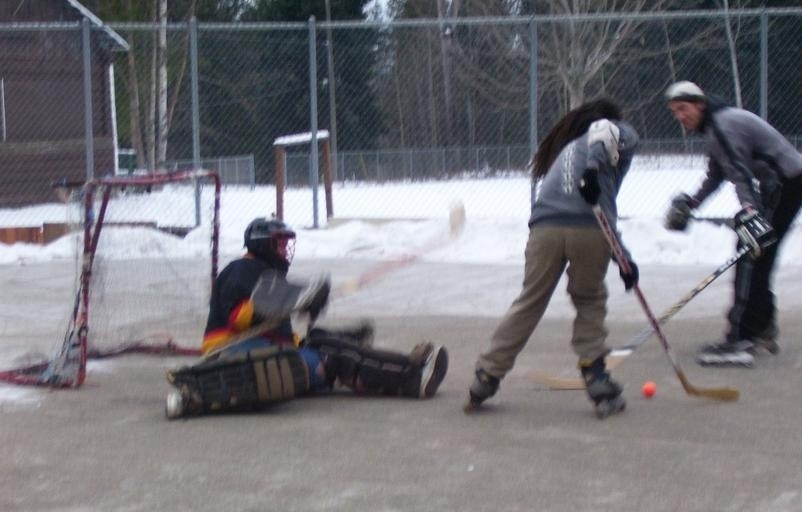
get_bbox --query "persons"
[166,212,449,419]
[661,80,802,367]
[465,98,641,419]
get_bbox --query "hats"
[664,81,705,105]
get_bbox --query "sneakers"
[166,392,184,418]
[419,344,448,398]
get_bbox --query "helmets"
[244,218,296,271]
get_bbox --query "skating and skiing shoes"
[695,338,755,368]
[465,369,499,410]
[749,326,781,353]
[581,357,625,418]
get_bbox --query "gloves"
[578,169,601,204]
[735,206,778,260]
[665,194,692,230]
[619,261,638,289]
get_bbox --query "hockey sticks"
[581,179,739,401]
[192,207,464,368]
[550,245,751,390]
